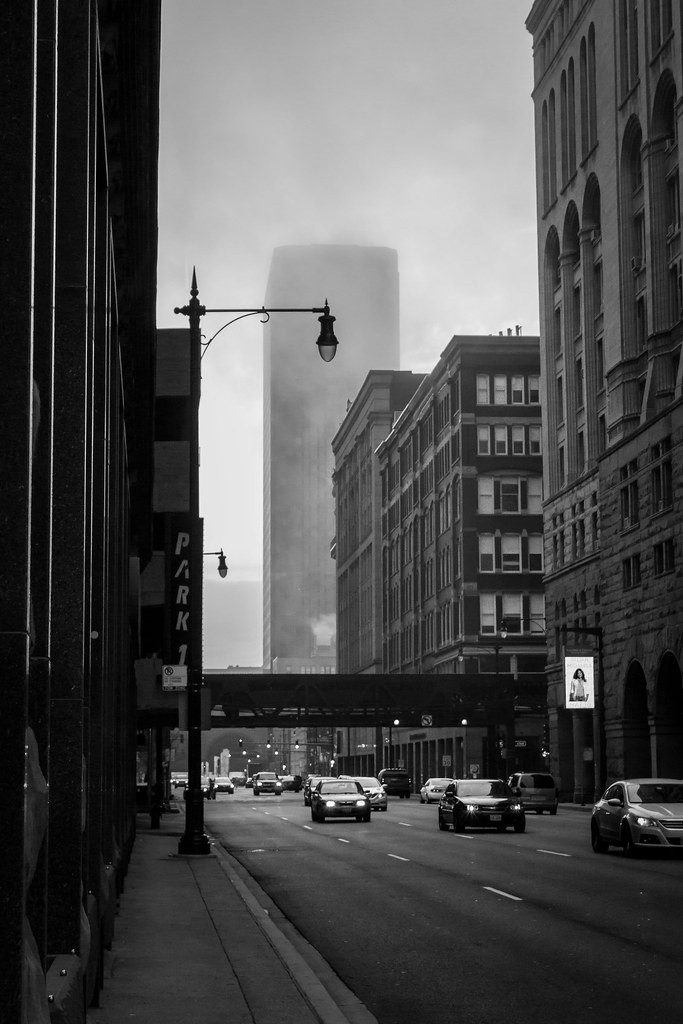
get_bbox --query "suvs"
[505,770,559,816]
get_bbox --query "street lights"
[455,642,508,779]
[178,265,342,853]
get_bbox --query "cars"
[438,777,526,834]
[589,777,683,857]
[171,767,456,823]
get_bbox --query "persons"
[570,669,590,701]
